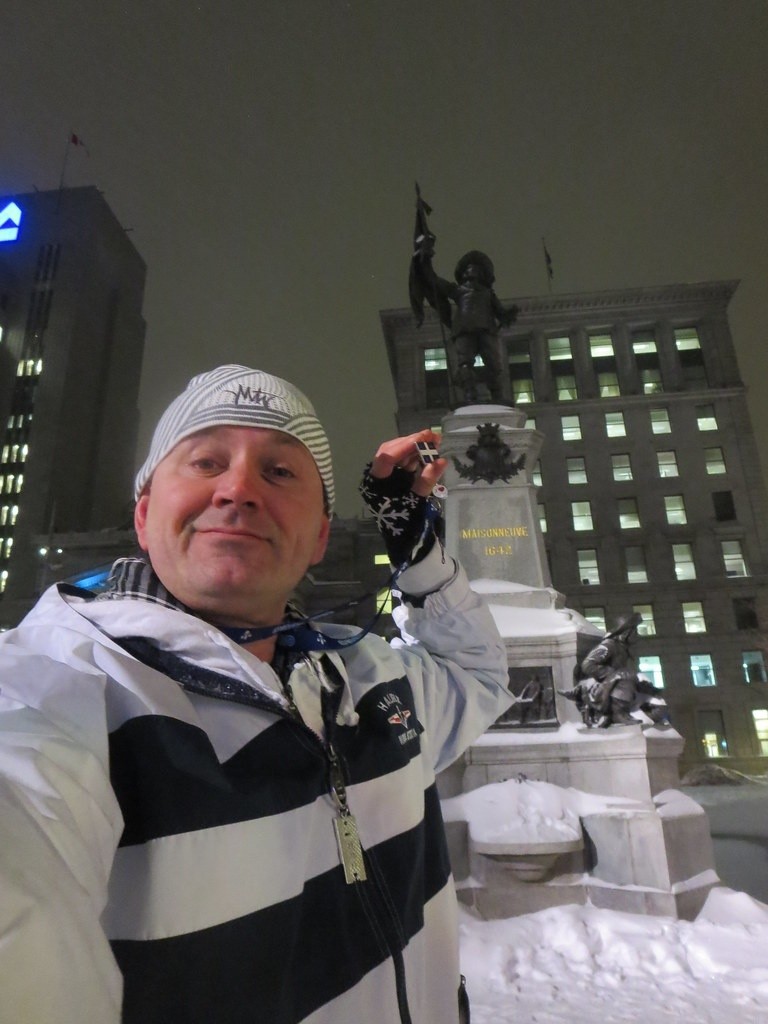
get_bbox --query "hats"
[137,365,337,525]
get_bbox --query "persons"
[0,364,517,1024]
[518,673,542,725]
[578,612,668,727]
[413,232,520,405]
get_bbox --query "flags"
[543,249,554,279]
